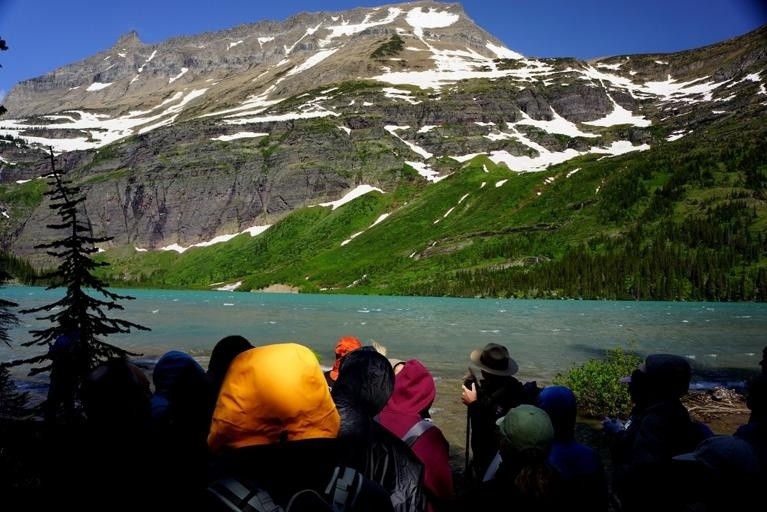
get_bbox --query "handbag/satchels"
[283,444,399,510]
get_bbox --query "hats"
[470,342,518,377]
[496,404,553,449]
[620,355,692,397]
[672,434,755,476]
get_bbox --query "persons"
[670,433,764,510]
[462,340,601,511]
[742,340,766,436]
[71,334,458,512]
[602,355,710,479]
